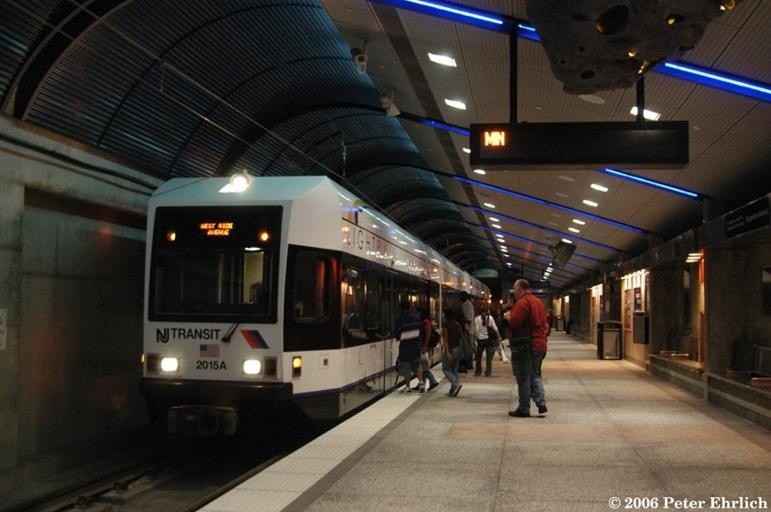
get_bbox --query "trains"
[142,169,492,435]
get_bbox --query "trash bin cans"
[597,321,622,360]
[556,316,564,331]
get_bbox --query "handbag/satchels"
[511,336,532,363]
[487,314,498,339]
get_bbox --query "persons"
[389,286,512,398]
[503,279,548,417]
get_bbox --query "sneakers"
[399,364,550,418]
